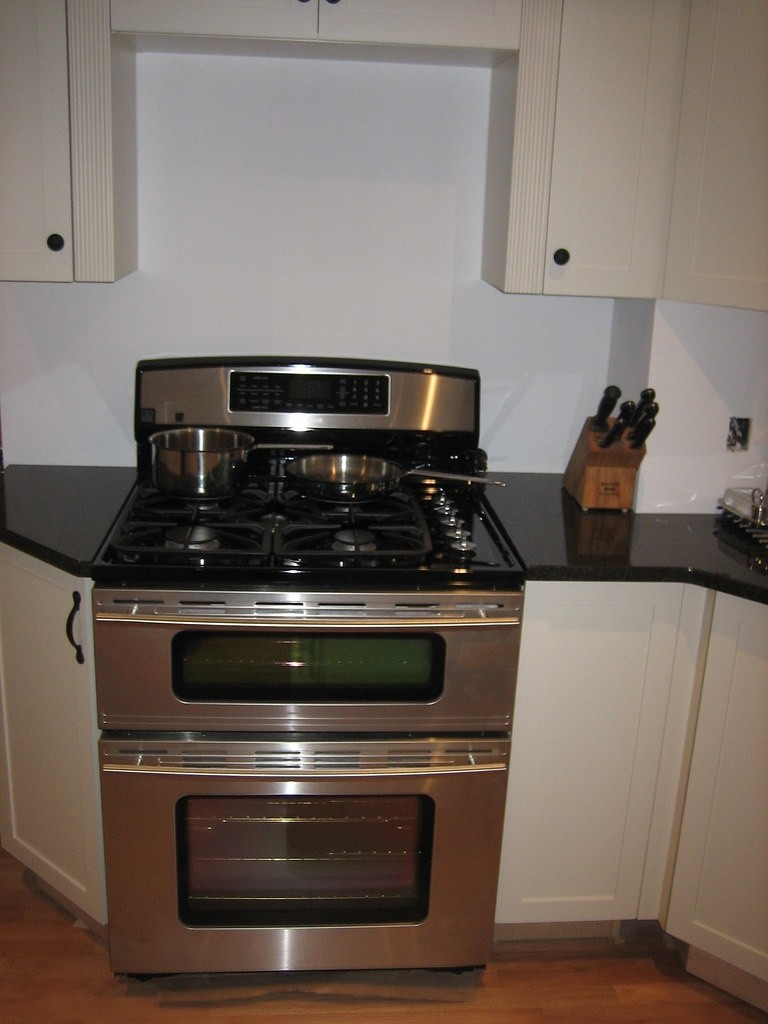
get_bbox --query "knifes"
[588,382,661,451]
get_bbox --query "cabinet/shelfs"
[0,536,111,944]
[1,0,140,286]
[480,0,768,316]
[109,0,521,71]
[494,580,768,1017]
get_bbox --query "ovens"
[94,593,524,975]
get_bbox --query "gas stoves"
[93,349,524,601]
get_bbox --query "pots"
[146,426,334,502]
[280,449,509,511]
